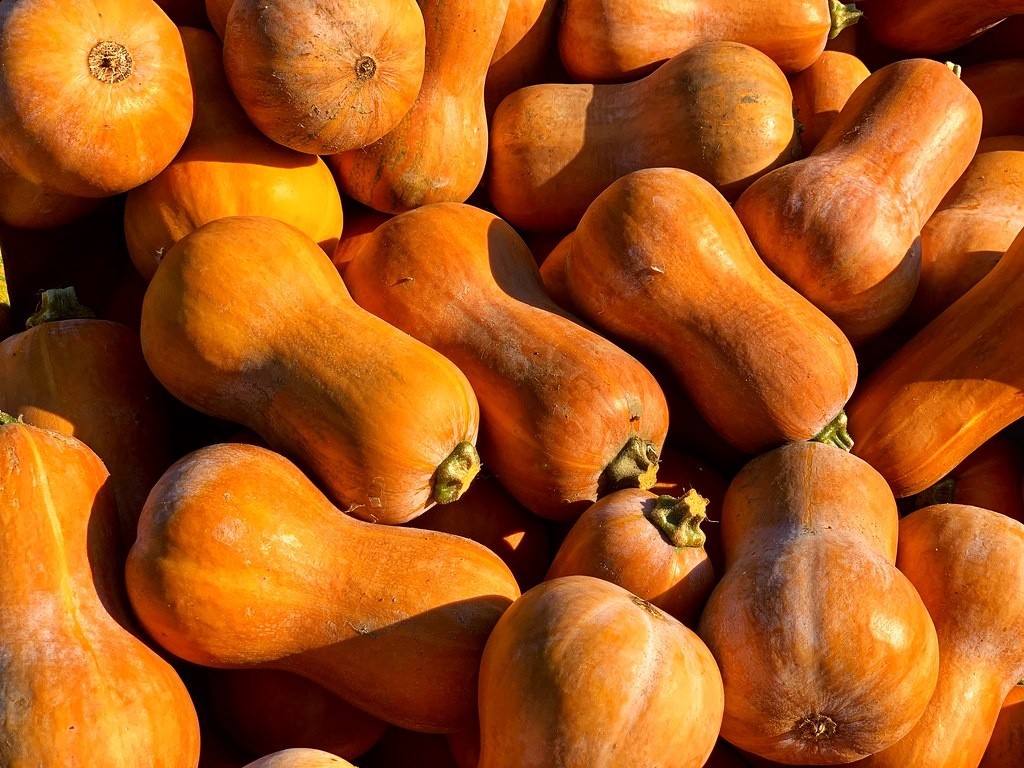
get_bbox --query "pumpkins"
[0,0,1024,768]
